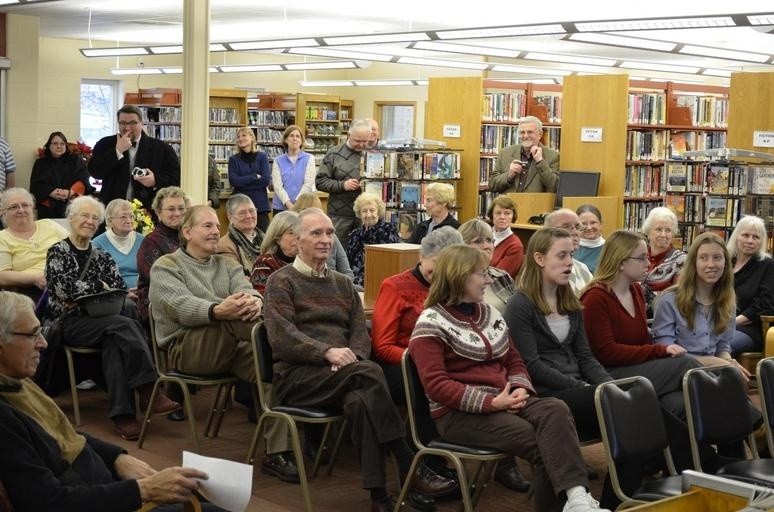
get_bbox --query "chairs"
[394,344,533,511]
[591,376,690,507]
[246,317,347,509]
[684,365,774,485]
[139,302,263,457]
[51,303,773,459]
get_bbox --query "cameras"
[517,161,529,175]
[131,167,147,179]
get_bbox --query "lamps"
[79,5,772,56]
[110,49,732,86]
[562,32,774,64]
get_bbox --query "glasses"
[3,201,30,214]
[626,254,650,264]
[554,224,584,235]
[118,119,139,128]
[110,213,136,223]
[5,325,45,343]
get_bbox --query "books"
[306,103,352,179]
[628,92,728,125]
[477,124,561,219]
[361,153,460,245]
[139,106,295,181]
[484,93,561,122]
[625,129,774,255]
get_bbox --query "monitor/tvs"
[553,170,601,210]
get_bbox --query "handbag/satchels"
[31,313,62,394]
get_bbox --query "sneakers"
[304,441,329,465]
[111,414,144,441]
[563,494,610,510]
[371,496,393,511]
[495,459,531,493]
[399,459,470,511]
[139,387,182,417]
[262,451,301,483]
[76,378,96,390]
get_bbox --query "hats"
[74,289,129,318]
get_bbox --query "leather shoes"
[168,411,185,421]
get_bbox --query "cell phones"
[358,176,366,181]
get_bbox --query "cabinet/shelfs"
[555,74,731,241]
[355,144,463,238]
[119,87,355,199]
[425,78,562,237]
[684,73,773,243]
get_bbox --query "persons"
[0,182,774,512]
[89,107,181,224]
[228,127,271,235]
[316,120,379,249]
[30,132,90,220]
[206,154,221,210]
[489,117,559,193]
[271,125,317,218]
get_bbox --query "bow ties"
[131,141,136,146]
